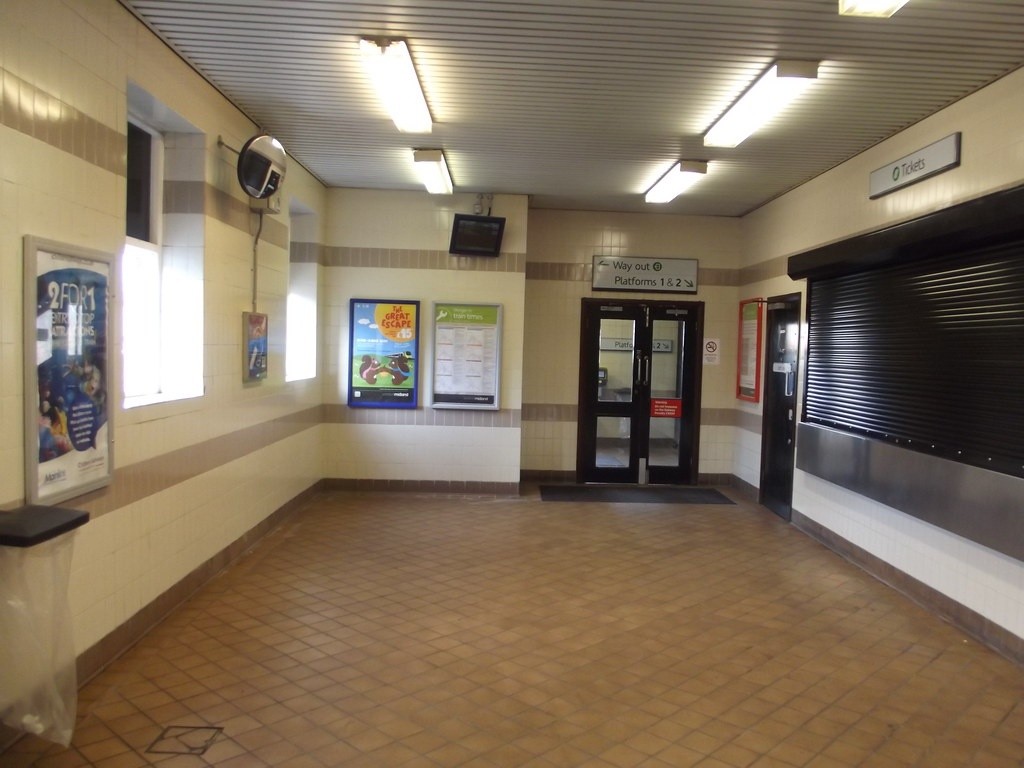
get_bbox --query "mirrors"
[236,133,289,200]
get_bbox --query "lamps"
[644,160,707,203]
[414,149,454,196]
[838,0,913,19]
[702,60,819,149]
[358,36,434,133]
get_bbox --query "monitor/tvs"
[449,213,506,257]
